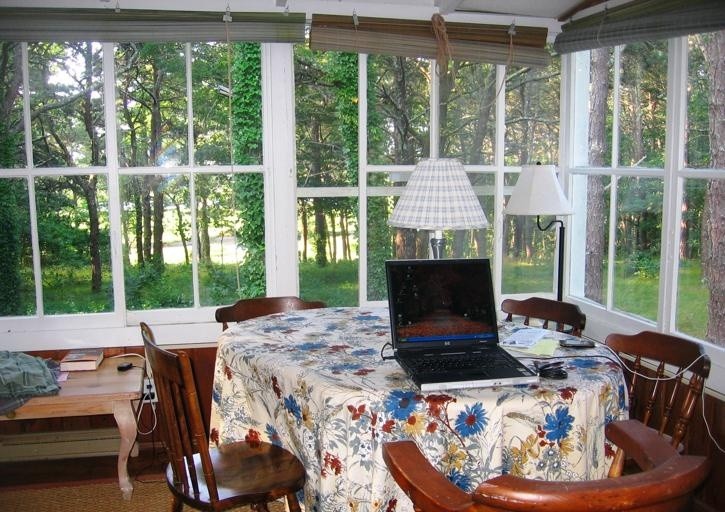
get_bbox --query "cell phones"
[117,361,132,371]
[559,339,595,348]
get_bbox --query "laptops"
[385,258,539,391]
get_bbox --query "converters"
[142,392,154,400]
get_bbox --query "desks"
[0,354,146,501]
[205,306,631,512]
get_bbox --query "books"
[59,349,104,370]
[499,328,548,348]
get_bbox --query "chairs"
[606,330,711,465]
[500,296,586,337]
[381,418,710,512]
[214,297,328,331]
[139,322,307,512]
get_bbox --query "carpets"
[0,476,290,512]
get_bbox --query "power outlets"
[142,376,159,403]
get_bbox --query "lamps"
[501,161,573,332]
[386,156,489,260]
